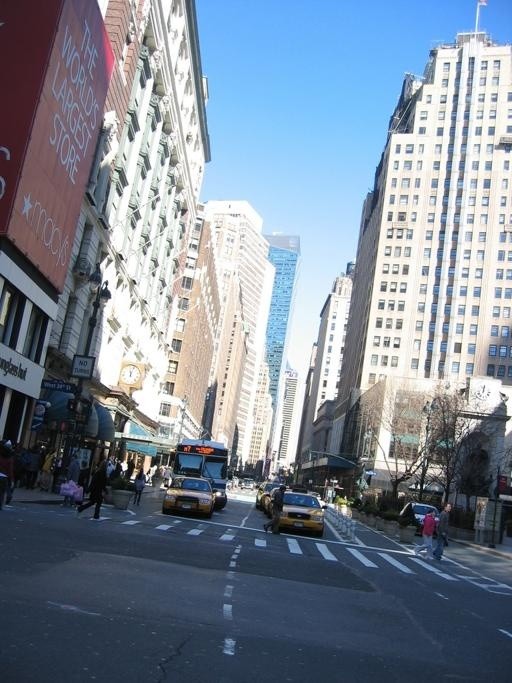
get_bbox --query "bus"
[170,438,228,511]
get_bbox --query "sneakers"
[75,507,80,520]
[94,517,104,522]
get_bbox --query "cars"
[233,476,255,491]
[163,476,217,519]
[400,501,440,538]
[256,481,327,537]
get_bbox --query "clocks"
[118,361,146,391]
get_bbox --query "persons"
[0,435,173,522]
[414,502,451,561]
[263,485,286,535]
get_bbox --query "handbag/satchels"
[60,480,84,502]
